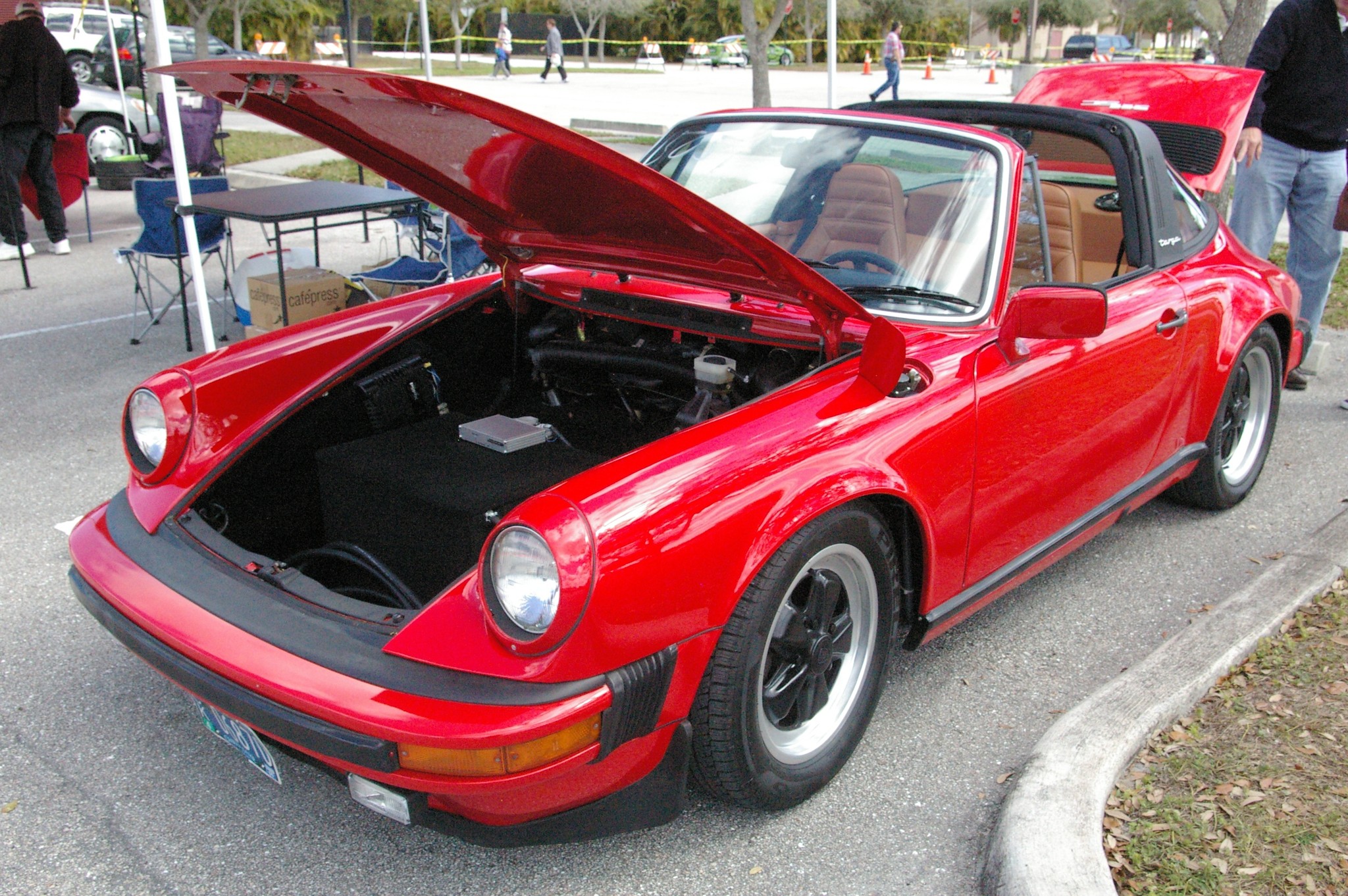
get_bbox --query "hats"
[15,0,44,18]
[891,20,901,31]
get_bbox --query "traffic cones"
[985,57,998,84]
[862,50,872,75]
[922,54,933,79]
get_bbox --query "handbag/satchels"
[502,43,513,54]
[550,53,561,68]
[1334,181,1348,231]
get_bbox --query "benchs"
[905,190,1134,282]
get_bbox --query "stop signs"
[1166,18,1173,32]
[1011,8,1020,24]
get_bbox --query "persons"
[0,0,80,261]
[538,19,568,83]
[1226,0,1348,390]
[491,21,513,80]
[868,21,904,102]
[1191,38,1206,63]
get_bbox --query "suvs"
[91,24,273,92]
[43,2,144,84]
[1063,34,1143,61]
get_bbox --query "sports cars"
[69,57,1314,846]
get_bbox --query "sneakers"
[0,241,35,260]
[47,238,71,254]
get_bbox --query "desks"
[163,180,426,352]
[19,133,94,244]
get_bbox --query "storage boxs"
[230,249,346,340]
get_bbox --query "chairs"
[113,176,240,346]
[1007,182,1084,304]
[795,163,908,276]
[140,91,230,179]
[350,179,497,302]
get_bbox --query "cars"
[708,34,794,67]
[57,80,162,176]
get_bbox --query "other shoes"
[869,94,875,101]
[1340,399,1348,409]
[1285,366,1308,388]
[488,76,497,79]
[536,78,544,82]
[560,78,569,82]
[503,75,512,80]
